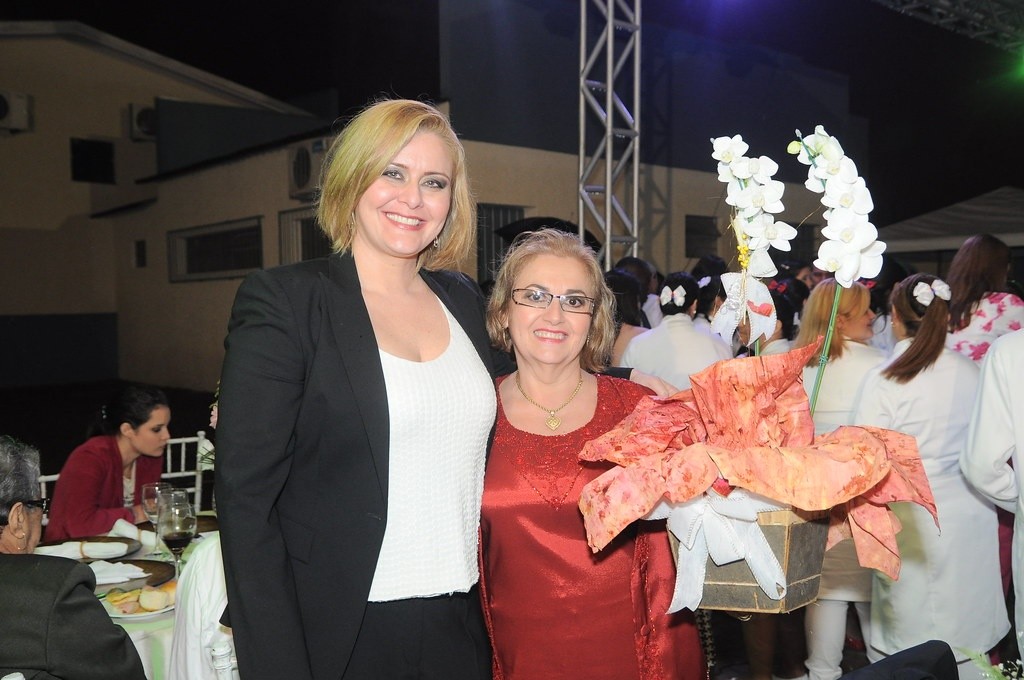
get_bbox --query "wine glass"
[141,482,172,560]
[157,489,197,583]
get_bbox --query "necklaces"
[513,370,583,431]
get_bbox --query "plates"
[98,596,175,620]
[36,536,142,564]
[86,559,175,596]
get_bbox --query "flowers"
[702,120,893,448]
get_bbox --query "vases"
[665,501,837,612]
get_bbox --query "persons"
[1,434,146,680]
[211,109,680,680]
[38,390,172,540]
[601,237,1024,680]
[481,229,703,680]
[961,327,1024,659]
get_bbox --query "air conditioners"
[0,88,29,129]
[130,102,159,141]
[286,138,329,197]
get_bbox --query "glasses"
[512,288,595,316]
[17,498,48,513]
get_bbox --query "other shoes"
[843,629,865,651]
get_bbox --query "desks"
[36,503,227,680]
